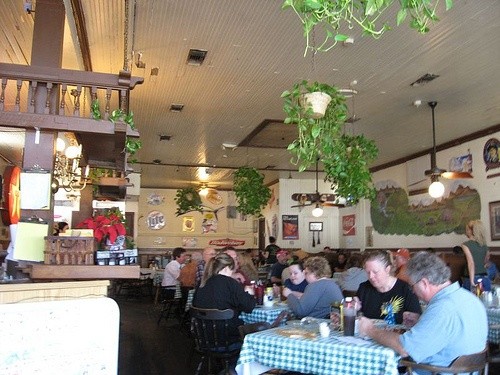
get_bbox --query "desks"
[238,290,295,331]
[234,317,414,375]
[127,265,158,298]
[149,268,166,302]
[483,304,500,344]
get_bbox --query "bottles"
[250,280,280,306]
[343,297,355,336]
[149,259,158,271]
[473,279,484,298]
[384,303,395,325]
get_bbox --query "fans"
[193,182,218,192]
[405,101,474,187]
[289,157,346,210]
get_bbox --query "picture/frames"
[489,200,500,241]
[309,221,323,232]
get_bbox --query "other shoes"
[220,369,236,375]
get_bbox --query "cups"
[481,291,492,307]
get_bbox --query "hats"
[392,249,410,257]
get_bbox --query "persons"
[262,236,280,264]
[193,253,256,375]
[484,250,497,281]
[161,247,193,314]
[339,253,369,291]
[195,247,216,285]
[274,262,308,301]
[221,246,252,288]
[358,251,488,375]
[355,252,422,325]
[323,246,347,279]
[282,256,343,320]
[461,220,491,294]
[53,222,69,236]
[390,248,411,282]
[176,252,202,286]
[270,252,311,286]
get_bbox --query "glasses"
[303,270,309,274]
[408,278,431,290]
[205,253,216,256]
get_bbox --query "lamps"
[53,130,91,207]
[427,175,446,199]
[311,204,323,218]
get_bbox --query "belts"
[465,273,487,278]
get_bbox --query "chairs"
[112,253,500,375]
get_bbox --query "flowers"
[73,213,128,245]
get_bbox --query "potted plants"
[231,166,272,220]
[280,78,351,176]
[174,186,204,218]
[320,131,379,207]
